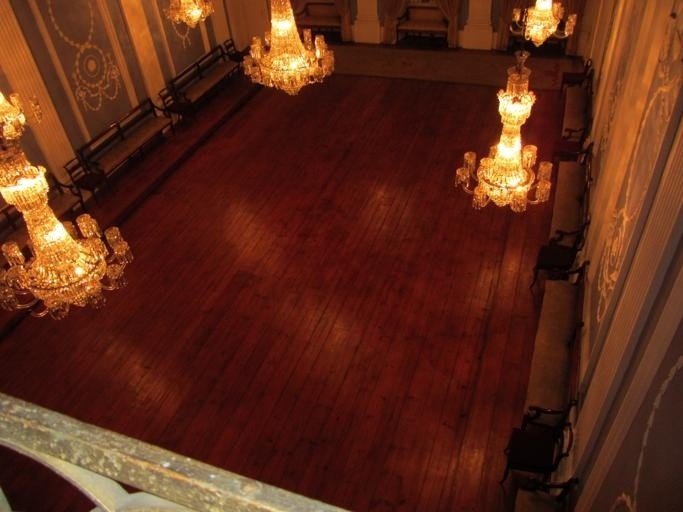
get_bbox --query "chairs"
[62,157,113,212]
[223,38,247,73]
[515,479,580,512]
[155,86,194,130]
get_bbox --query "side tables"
[565,142,583,159]
[529,246,579,288]
[502,418,574,481]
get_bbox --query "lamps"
[162,0,216,31]
[506,1,588,44]
[452,1,562,215]
[0,127,133,320]
[0,86,42,144]
[238,0,335,98]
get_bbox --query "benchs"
[519,260,586,424]
[558,62,591,138]
[76,98,174,195]
[546,153,590,245]
[392,7,449,44]
[0,171,85,242]
[157,45,239,115]
[292,2,344,38]
